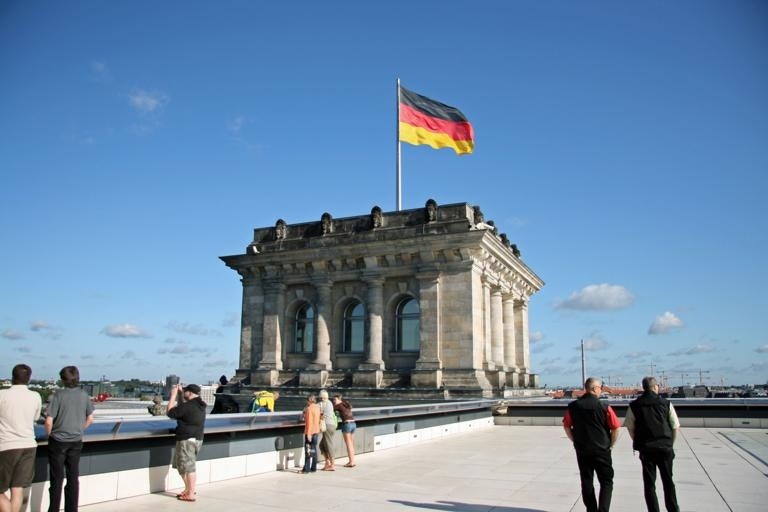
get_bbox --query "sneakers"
[298,470,309,474]
[323,463,336,471]
[344,462,355,466]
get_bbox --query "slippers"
[177,491,196,501]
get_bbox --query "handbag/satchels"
[319,415,327,432]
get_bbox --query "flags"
[397,84,474,156]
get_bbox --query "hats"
[182,384,201,395]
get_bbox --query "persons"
[166,381,207,503]
[296,393,321,475]
[42,364,95,512]
[247,390,279,426]
[560,377,621,512]
[622,375,680,512]
[0,362,42,512]
[332,393,357,468]
[317,389,338,472]
